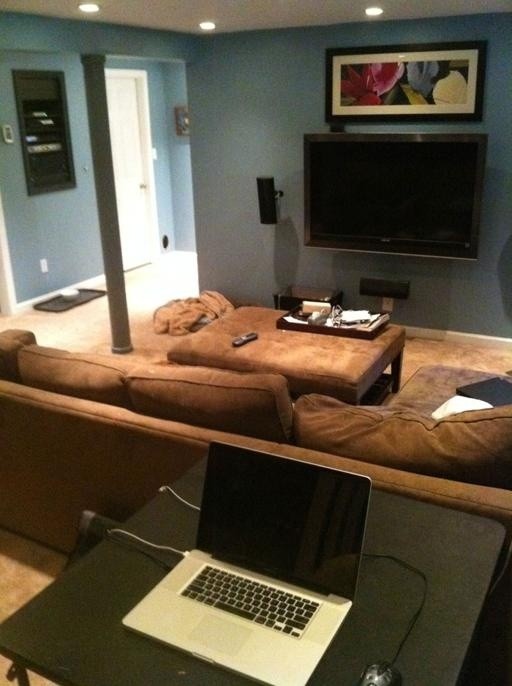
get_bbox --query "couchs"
[1,329,512,686]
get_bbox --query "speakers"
[359,277,409,300]
[256,176,278,224]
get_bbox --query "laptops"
[121,441,372,686]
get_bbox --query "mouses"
[358,660,401,686]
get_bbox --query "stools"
[272,284,344,310]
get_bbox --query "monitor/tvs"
[303,133,489,261]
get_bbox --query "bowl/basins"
[58,288,80,302]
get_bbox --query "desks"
[0,449,505,686]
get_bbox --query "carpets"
[33,288,107,312]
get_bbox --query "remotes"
[232,332,257,346]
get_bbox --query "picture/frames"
[324,39,487,122]
